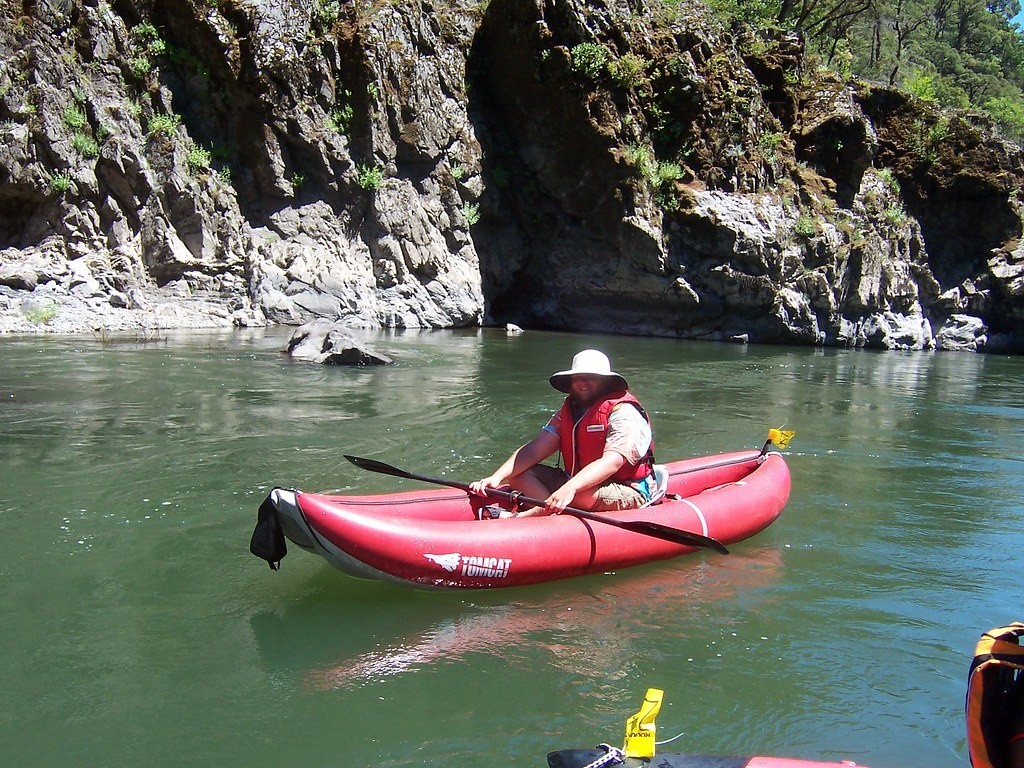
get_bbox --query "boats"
[247,426,795,590]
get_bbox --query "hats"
[549,349,631,395]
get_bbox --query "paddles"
[343,453,731,556]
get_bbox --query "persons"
[469,348,659,523]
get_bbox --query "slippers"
[477,505,523,522]
